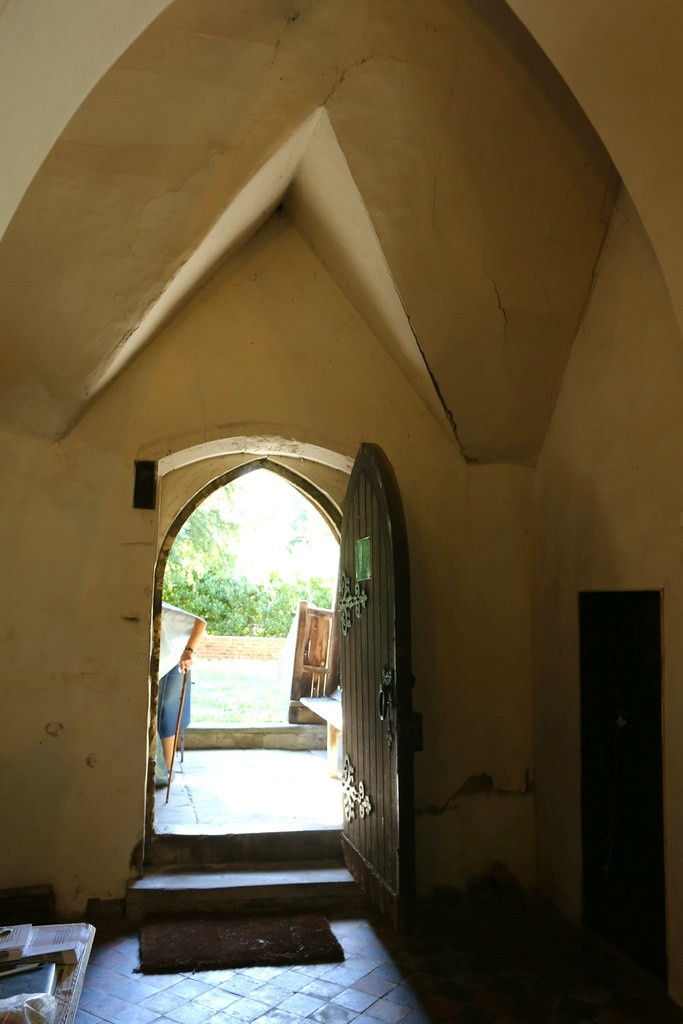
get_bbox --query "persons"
[157,602,207,783]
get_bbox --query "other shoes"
[164,769,175,785]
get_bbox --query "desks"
[0,923,97,1024]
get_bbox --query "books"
[0,923,93,964]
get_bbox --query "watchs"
[184,648,193,652]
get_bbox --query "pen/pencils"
[0,961,45,976]
[0,930,11,937]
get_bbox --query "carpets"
[133,910,346,975]
